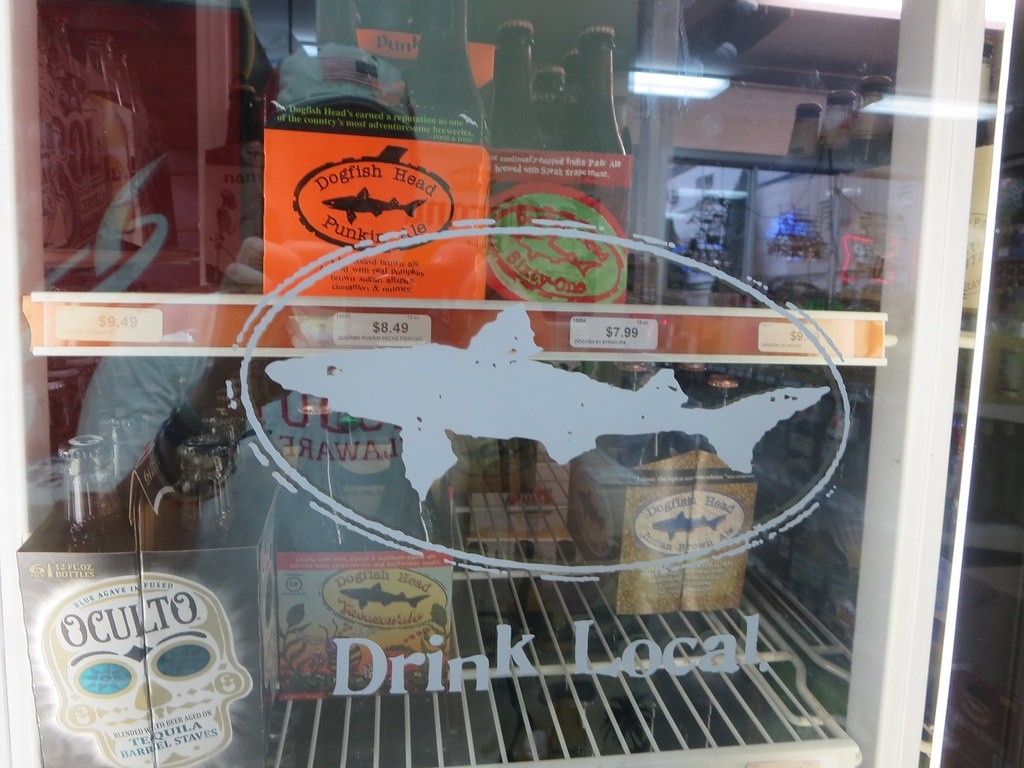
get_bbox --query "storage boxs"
[563,440,757,617]
[16,499,156,768]
[136,478,277,768]
[275,473,455,702]
[485,126,633,302]
[262,95,494,300]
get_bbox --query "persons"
[62,44,483,684]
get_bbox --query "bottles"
[176,435,245,553]
[785,73,898,194]
[248,537,791,768]
[46,356,105,441]
[28,11,163,252]
[97,406,146,517]
[313,0,629,159]
[606,360,667,469]
[701,374,745,463]
[199,406,251,514]
[57,434,135,556]
[668,359,713,463]
[975,33,997,147]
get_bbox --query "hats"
[262,43,417,141]
